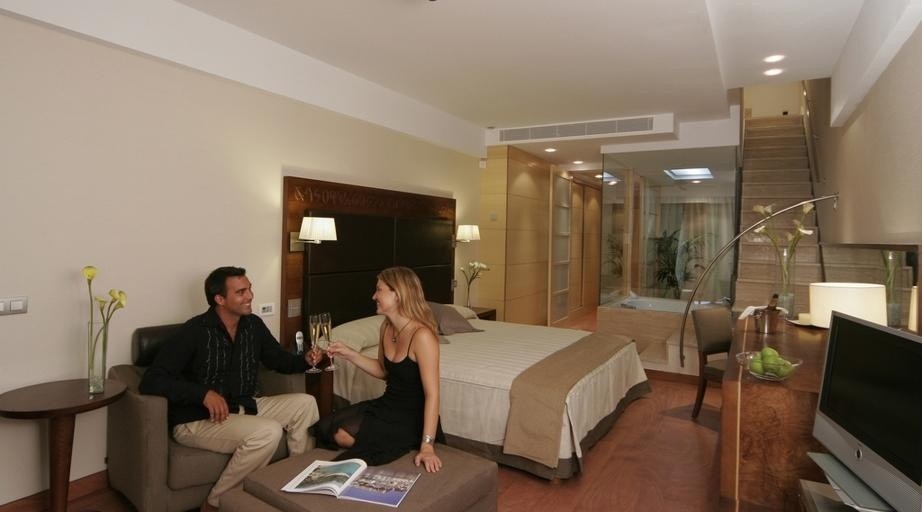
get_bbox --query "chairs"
[690,304,733,419]
[107,323,306,512]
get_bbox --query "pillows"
[315,314,386,353]
[427,304,480,334]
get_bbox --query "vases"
[464,283,472,308]
[772,247,799,318]
[86,319,108,394]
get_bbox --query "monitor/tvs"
[806,310,922,512]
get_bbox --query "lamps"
[810,282,888,328]
[290,216,338,252]
[451,224,480,249]
[908,287,918,331]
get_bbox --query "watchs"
[421,435,435,447]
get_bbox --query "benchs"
[220,442,499,512]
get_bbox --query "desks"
[720,310,828,511]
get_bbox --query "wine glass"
[318,312,344,372]
[305,315,323,373]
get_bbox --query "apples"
[748,347,793,377]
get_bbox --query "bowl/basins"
[735,350,804,383]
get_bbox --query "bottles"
[763,293,778,310]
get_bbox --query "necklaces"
[391,318,412,344]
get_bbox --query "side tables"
[0,376,128,511]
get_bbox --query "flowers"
[81,265,127,387]
[751,202,815,313]
[457,261,490,307]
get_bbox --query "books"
[280,459,422,508]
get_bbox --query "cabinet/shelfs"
[477,145,549,326]
[570,179,601,310]
[547,164,573,326]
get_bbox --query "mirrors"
[819,242,919,334]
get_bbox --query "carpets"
[1,369,724,511]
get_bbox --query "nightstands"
[461,305,496,320]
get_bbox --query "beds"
[304,206,653,487]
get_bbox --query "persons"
[138,266,324,512]
[324,266,447,474]
